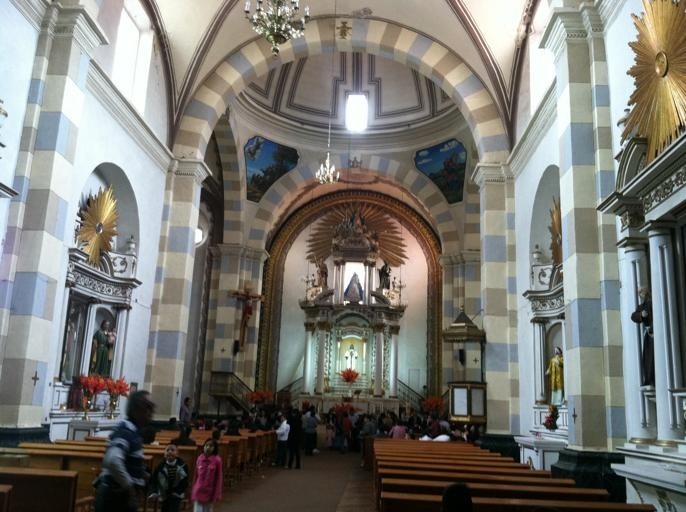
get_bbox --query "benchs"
[0,425,281,512]
[369,436,656,512]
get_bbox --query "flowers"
[244,390,274,403]
[79,374,130,399]
[340,368,359,383]
[421,395,445,418]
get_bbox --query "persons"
[93,391,154,511]
[170,396,481,469]
[188,437,225,511]
[630,285,655,386]
[545,346,564,405]
[145,442,190,511]
[89,319,112,377]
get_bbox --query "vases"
[81,396,119,421]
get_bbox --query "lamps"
[315,76,340,184]
[244,0,310,55]
[531,243,542,266]
[344,52,368,134]
[126,235,139,258]
